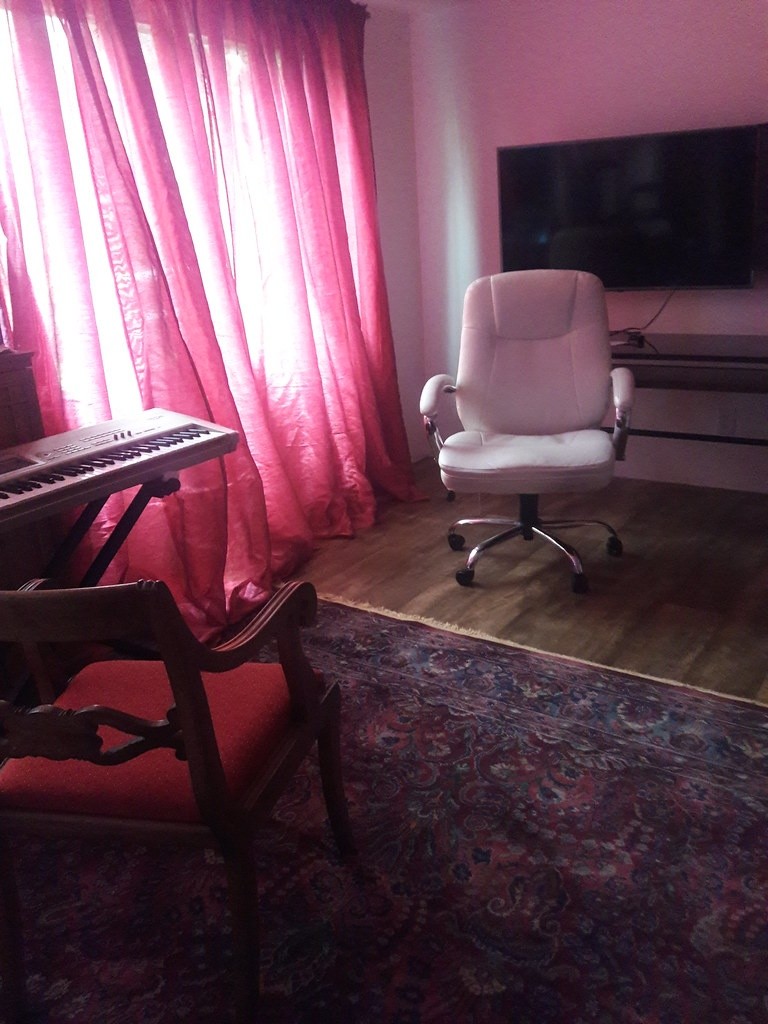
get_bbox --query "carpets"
[0,582,767,1023]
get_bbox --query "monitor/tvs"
[496,123,753,291]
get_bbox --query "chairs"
[1,577,355,1024]
[419,269,635,594]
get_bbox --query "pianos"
[0,406,236,587]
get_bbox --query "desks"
[609,330,768,448]
